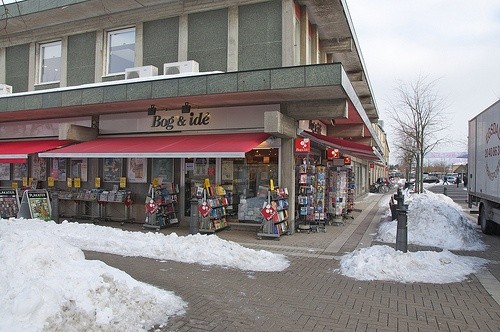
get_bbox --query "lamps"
[182,102,190,113]
[148,105,157,115]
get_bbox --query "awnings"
[38,132,272,159]
[0,140,71,163]
[296,127,385,163]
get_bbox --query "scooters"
[369,178,392,194]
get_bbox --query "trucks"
[465,99,500,236]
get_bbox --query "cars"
[443,176,457,184]
[423,176,440,184]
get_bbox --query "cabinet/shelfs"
[256,187,290,240]
[142,193,180,233]
[198,195,233,235]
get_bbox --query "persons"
[455,177,459,188]
[442,177,448,186]
[463,174,468,187]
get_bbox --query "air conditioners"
[125,65,158,80]
[163,60,199,75]
[0,84,12,95]
